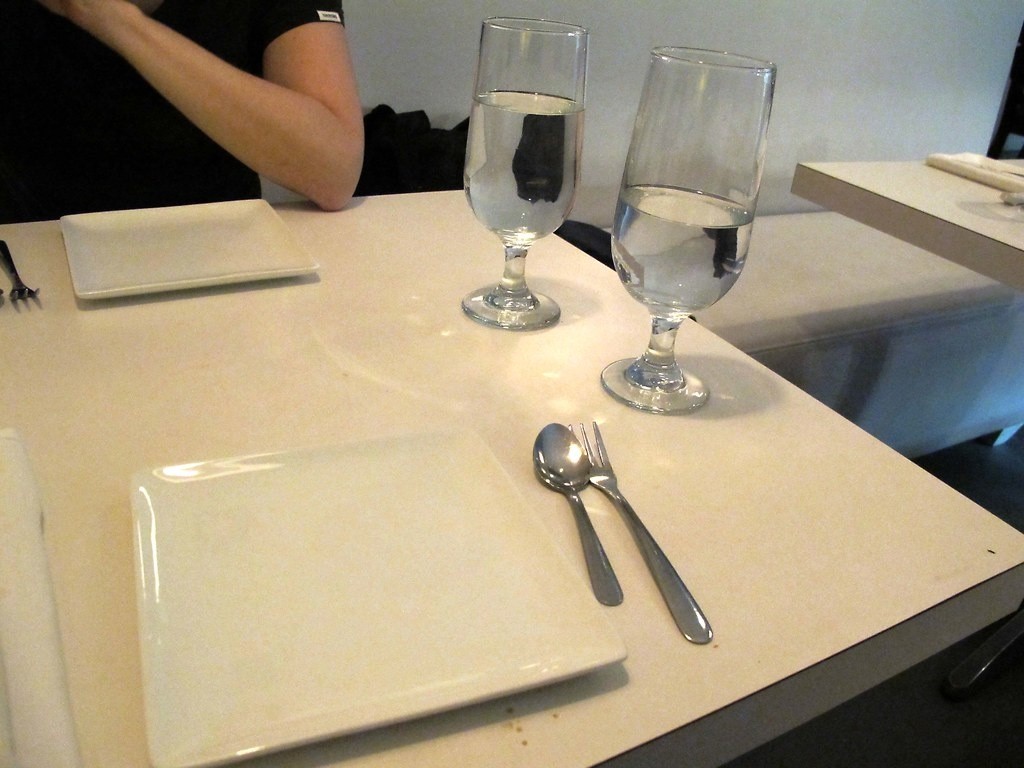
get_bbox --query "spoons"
[533,422,624,605]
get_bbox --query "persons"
[1,1,365,222]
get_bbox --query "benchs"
[605,211,1024,458]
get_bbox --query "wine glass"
[460,16,589,331]
[601,47,777,417]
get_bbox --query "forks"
[0,239,40,300]
[565,422,713,645]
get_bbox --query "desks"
[790,158,1024,293]
[0,189,1024,768]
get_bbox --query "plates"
[59,199,319,299]
[127,428,629,768]
